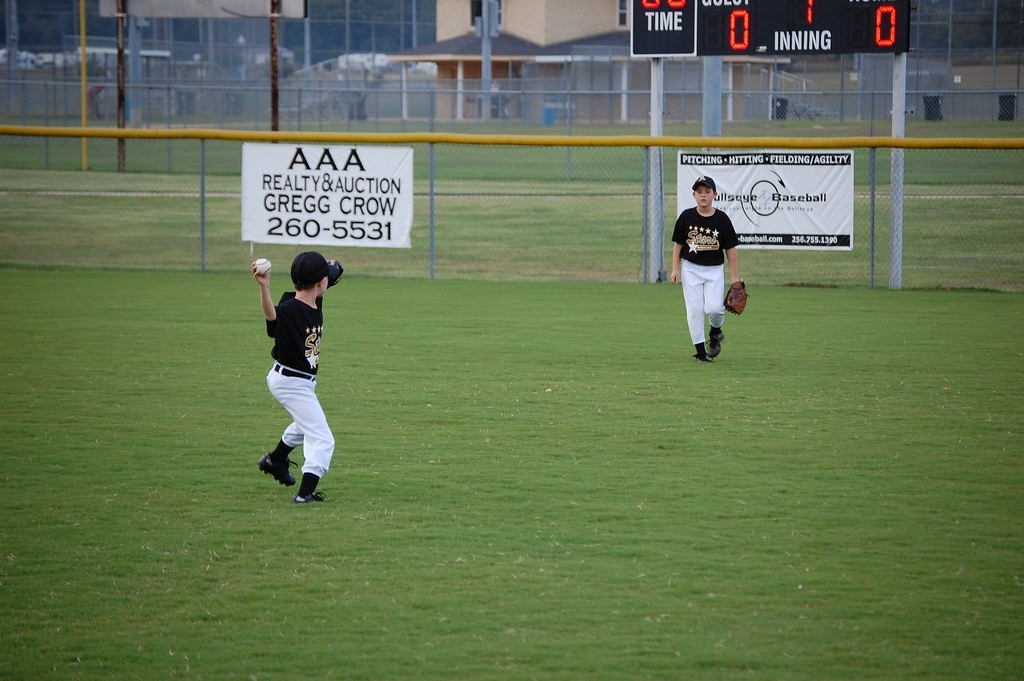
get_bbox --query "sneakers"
[293,492,327,503]
[693,353,714,362]
[707,325,724,358]
[258,452,298,487]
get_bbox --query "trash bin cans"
[772,97,790,120]
[922,95,944,121]
[998,95,1016,121]
[540,106,554,125]
[176,89,195,115]
[348,90,367,120]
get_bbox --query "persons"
[671,175,747,361]
[251,251,345,503]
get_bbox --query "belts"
[274,364,315,382]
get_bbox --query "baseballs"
[254,258,272,275]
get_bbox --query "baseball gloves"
[723,280,751,316]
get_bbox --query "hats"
[692,176,716,192]
[291,252,334,286]
